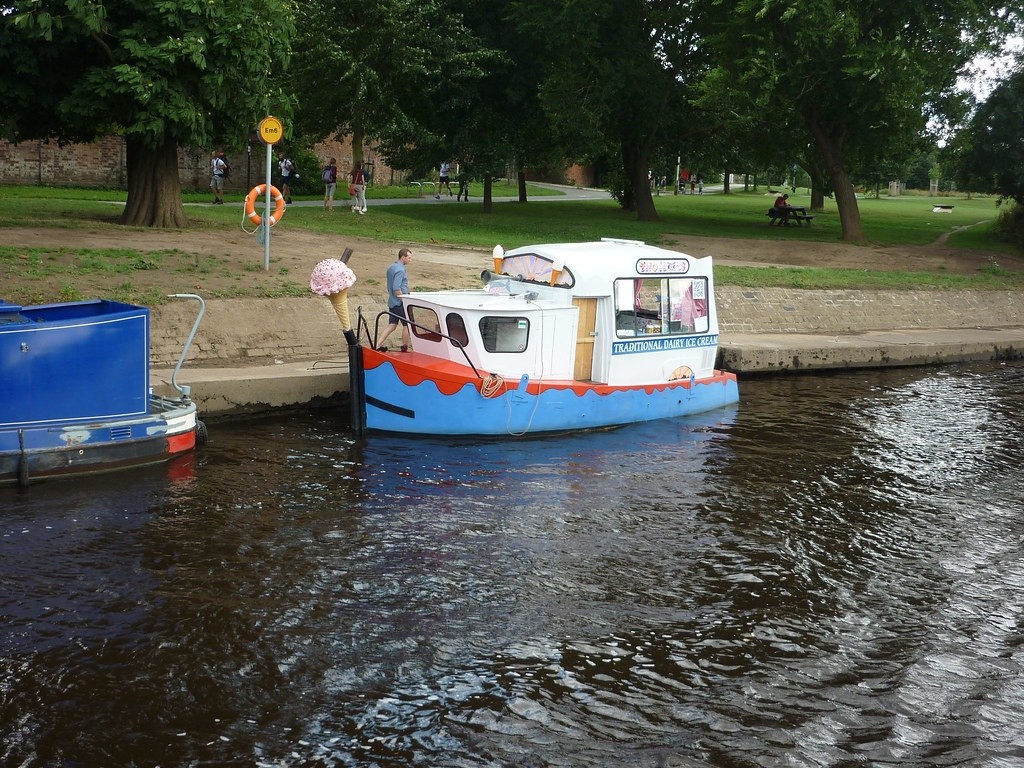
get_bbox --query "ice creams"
[550,260,563,286]
[310,257,357,332]
[493,245,503,274]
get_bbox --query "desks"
[779,206,811,226]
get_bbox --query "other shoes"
[213,197,223,204]
[283,197,292,204]
[359,211,365,214]
[435,195,440,199]
[351,206,359,212]
[450,191,452,197]
[464,198,469,202]
[457,198,461,202]
[362,207,367,212]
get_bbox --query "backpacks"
[216,153,233,185]
[282,159,296,188]
[322,165,333,184]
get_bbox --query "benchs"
[765,213,813,226]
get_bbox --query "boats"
[0,294,209,487]
[310,238,740,431]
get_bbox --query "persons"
[376,249,413,352]
[321,158,337,211]
[690,178,696,194]
[454,168,472,202]
[210,151,228,204]
[699,181,704,195]
[774,194,788,207]
[435,162,452,199]
[679,178,685,190]
[346,161,369,214]
[277,152,294,205]
[661,176,667,192]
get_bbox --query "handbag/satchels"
[350,184,356,196]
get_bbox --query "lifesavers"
[244,183,285,226]
